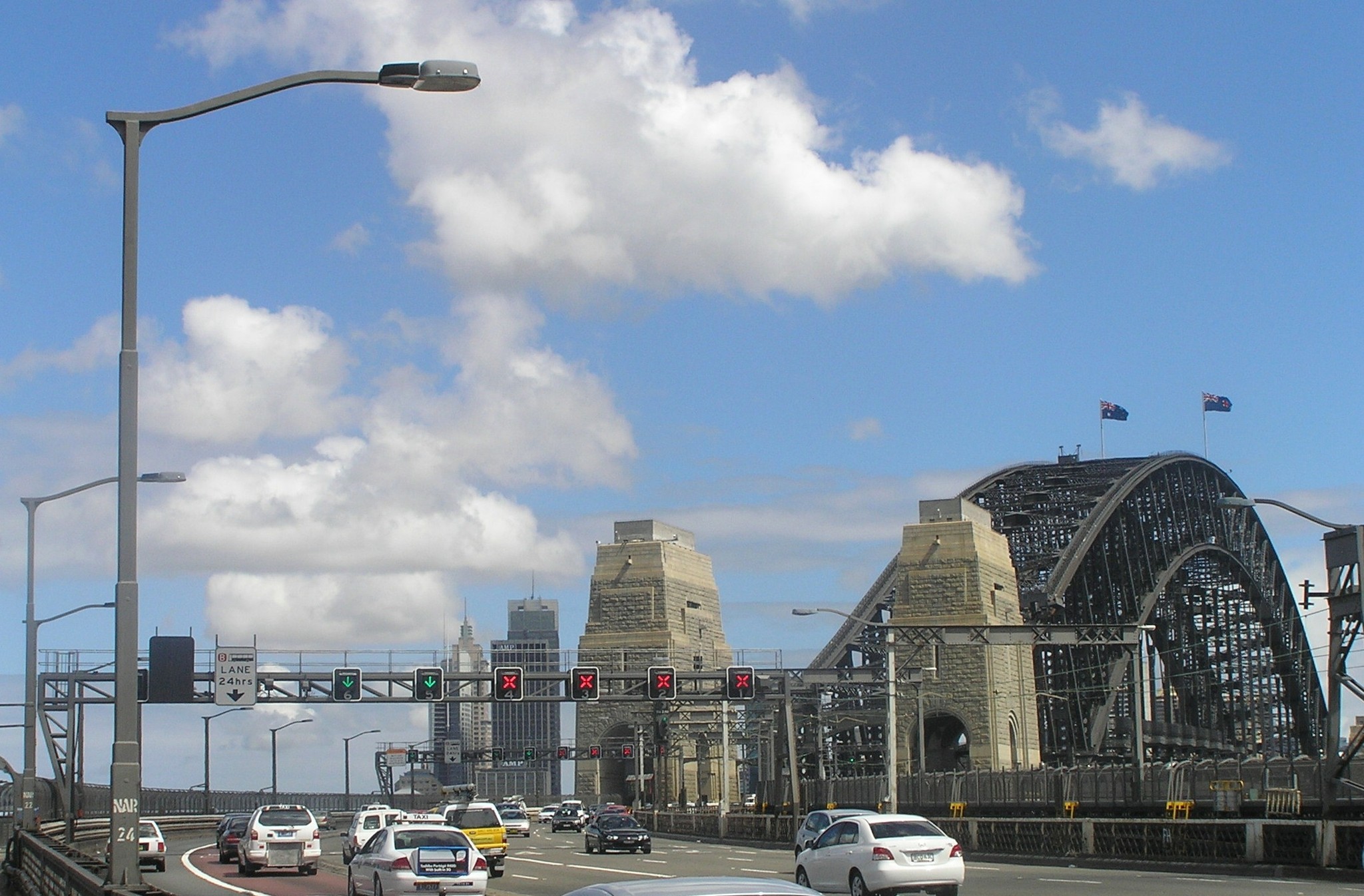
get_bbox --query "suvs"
[236,804,322,876]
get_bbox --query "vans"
[432,783,510,877]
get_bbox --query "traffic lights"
[647,665,676,701]
[658,713,669,737]
[523,746,536,761]
[491,747,504,762]
[493,666,524,702]
[571,666,600,701]
[557,745,569,761]
[621,744,635,759]
[407,749,418,763]
[589,744,602,760]
[414,666,444,701]
[332,667,363,702]
[726,665,756,700]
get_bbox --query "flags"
[1202,392,1233,412]
[1101,399,1129,421]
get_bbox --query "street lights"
[102,58,484,896]
[916,667,937,774]
[407,737,447,811]
[342,729,382,812]
[20,469,187,831]
[268,718,313,805]
[792,605,899,815]
[199,706,255,815]
[1214,496,1364,818]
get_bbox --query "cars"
[339,794,632,867]
[794,814,966,896]
[217,815,252,864]
[347,813,489,895]
[793,808,880,864]
[103,820,167,873]
[562,877,823,896]
[583,813,652,855]
[215,811,252,848]
[311,810,338,830]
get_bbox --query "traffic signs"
[213,646,258,707]
[443,739,462,764]
